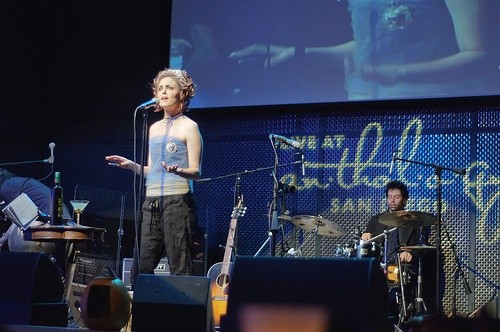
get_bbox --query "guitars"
[207,199,247,328]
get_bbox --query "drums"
[348,238,380,260]
[388,281,410,320]
[385,262,409,286]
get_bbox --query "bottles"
[336,239,358,256]
[50,172,63,225]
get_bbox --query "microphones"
[301,154,306,176]
[139,97,159,108]
[49,142,58,164]
[272,134,301,149]
[389,152,396,173]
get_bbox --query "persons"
[0,166,76,312]
[360,180,422,332]
[105,69,205,290]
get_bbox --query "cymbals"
[290,215,345,237]
[400,244,437,251]
[260,214,291,221]
[378,210,437,228]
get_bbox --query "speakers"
[131,274,216,332]
[223,255,394,332]
[0,251,70,330]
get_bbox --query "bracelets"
[177,168,184,174]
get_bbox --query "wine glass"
[69,200,89,227]
